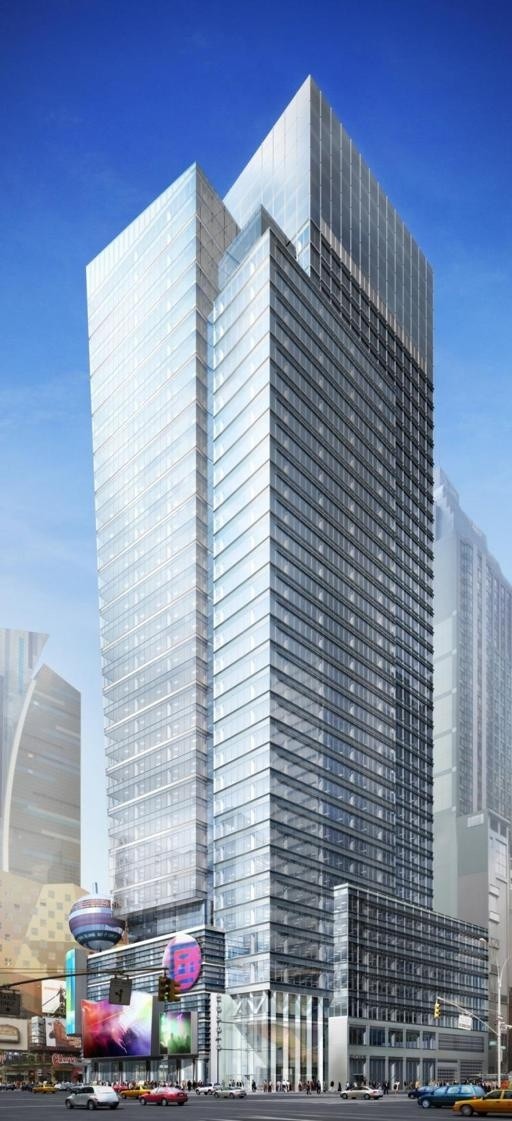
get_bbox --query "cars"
[195,1083,222,1095]
[340,1086,383,1100]
[65,1086,120,1109]
[121,1085,151,1099]
[407,1085,437,1099]
[418,1085,485,1109]
[0,1081,129,1094]
[453,1089,512,1117]
[213,1087,247,1099]
[138,1087,188,1106]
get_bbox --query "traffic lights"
[159,976,182,1003]
[434,1004,441,1018]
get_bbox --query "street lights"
[479,937,511,1088]
